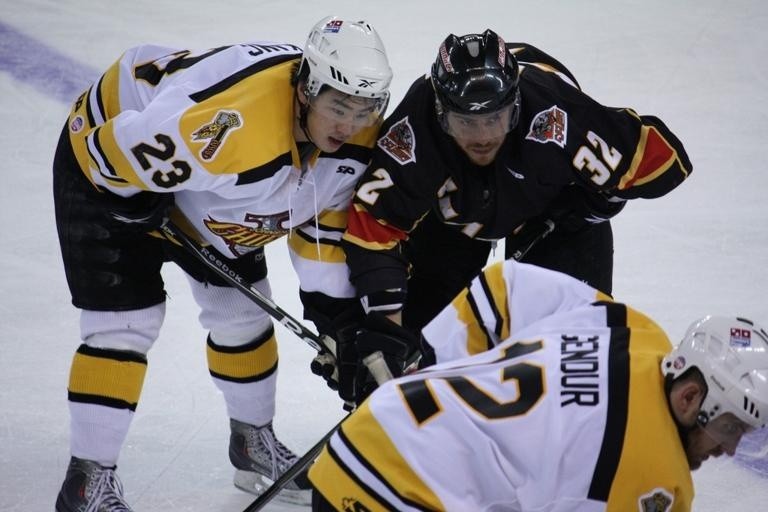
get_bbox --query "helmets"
[429,28,522,140]
[659,311,767,429]
[296,15,394,127]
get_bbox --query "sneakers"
[228,416,314,491]
[55,455,134,512]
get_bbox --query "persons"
[296,251,768,511]
[47,14,394,511]
[339,26,695,411]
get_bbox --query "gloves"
[583,187,628,225]
[104,188,175,234]
[308,304,425,414]
